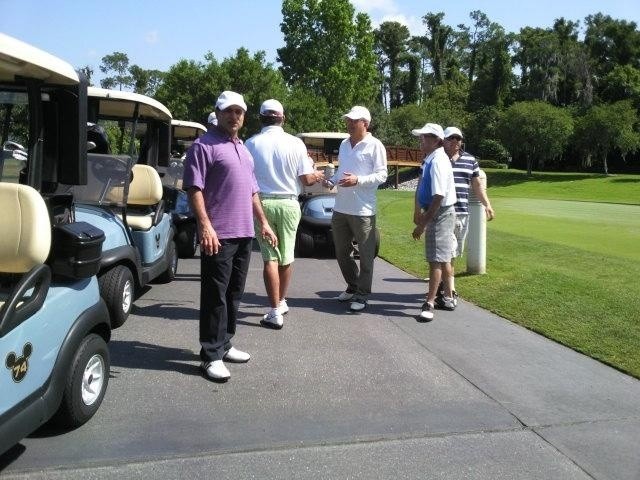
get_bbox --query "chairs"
[0,158,165,338]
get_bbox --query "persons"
[324,106,388,312]
[176,148,193,218]
[411,122,458,321]
[308,151,315,169]
[414,124,494,311]
[324,160,335,177]
[244,96,325,328]
[193,111,218,145]
[182,90,278,380]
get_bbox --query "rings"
[321,177,323,180]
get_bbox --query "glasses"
[446,135,462,141]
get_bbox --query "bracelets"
[314,174,319,182]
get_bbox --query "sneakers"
[223,346,251,363]
[263,302,289,328]
[351,298,368,311]
[338,291,357,301]
[435,280,458,311]
[419,297,435,321]
[200,359,231,382]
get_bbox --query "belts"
[260,193,297,200]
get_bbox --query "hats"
[412,122,445,141]
[444,127,463,139]
[341,106,371,122]
[259,99,284,117]
[215,90,248,112]
[208,112,218,126]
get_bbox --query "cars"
[3,140,25,159]
